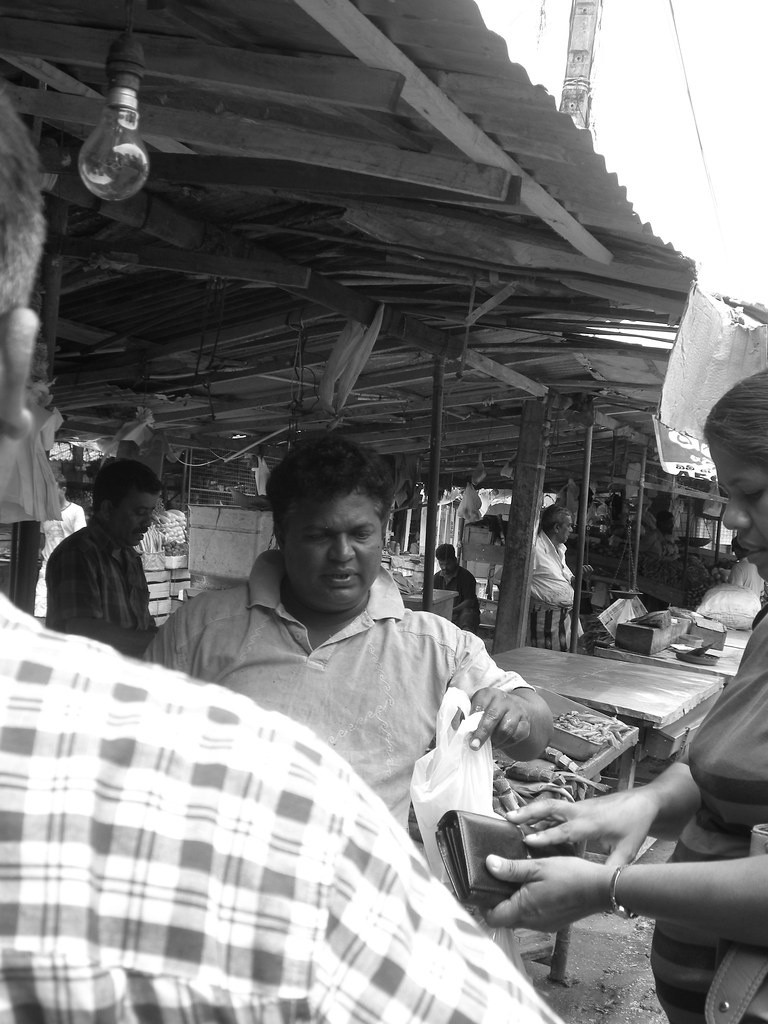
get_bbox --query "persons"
[480,369,768,1024]
[0,88,566,1024]
[140,436,555,836]
[434,543,480,631]
[528,504,594,653]
[728,536,765,602]
[34,472,88,618]
[635,510,674,571]
[45,458,164,653]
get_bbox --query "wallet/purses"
[434,808,576,908]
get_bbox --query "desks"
[490,643,745,987]
[406,590,462,621]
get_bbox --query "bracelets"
[609,865,638,920]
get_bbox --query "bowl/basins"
[678,633,704,647]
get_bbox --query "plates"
[676,651,719,665]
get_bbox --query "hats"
[731,536,740,552]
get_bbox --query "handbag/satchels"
[702,481,722,517]
[457,484,483,523]
[251,456,271,495]
[409,688,525,978]
